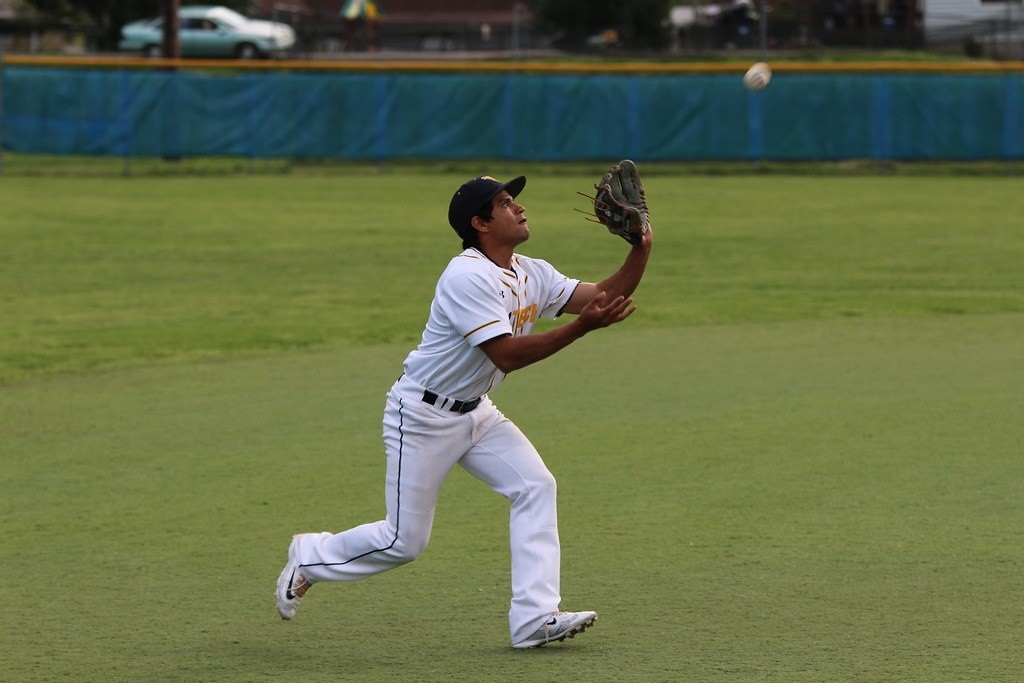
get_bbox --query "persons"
[274,159,653,650]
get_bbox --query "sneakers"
[275,535,312,620]
[514,610,598,648]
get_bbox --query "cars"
[117,6,298,61]
[325,1,923,53]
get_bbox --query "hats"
[448,176,527,238]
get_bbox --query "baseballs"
[743,69,768,91]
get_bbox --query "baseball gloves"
[575,159,651,247]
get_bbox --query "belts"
[398,373,482,416]
